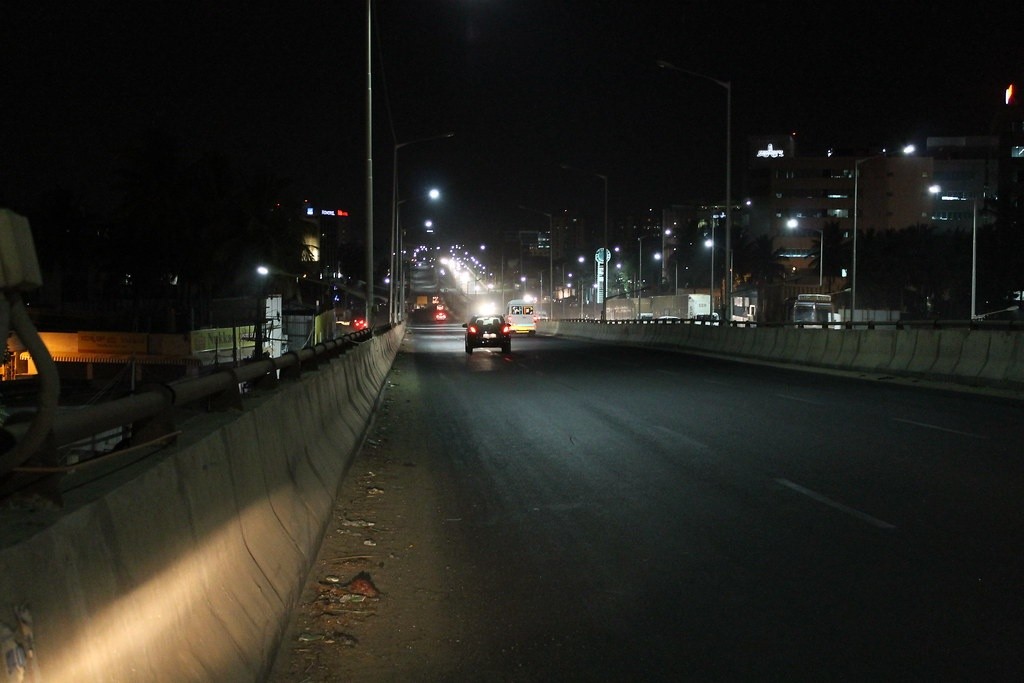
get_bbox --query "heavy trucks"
[606,294,756,328]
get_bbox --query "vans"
[503,299,536,336]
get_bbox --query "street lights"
[850,144,916,323]
[709,204,743,327]
[926,183,978,320]
[786,218,824,294]
[638,229,671,320]
[517,204,554,321]
[656,59,733,323]
[389,132,455,324]
[562,258,585,318]
[555,163,608,321]
[398,220,433,321]
[393,189,440,325]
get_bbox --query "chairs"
[477,320,483,325]
[493,319,499,325]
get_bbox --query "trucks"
[784,293,837,330]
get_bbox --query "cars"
[461,313,512,355]
